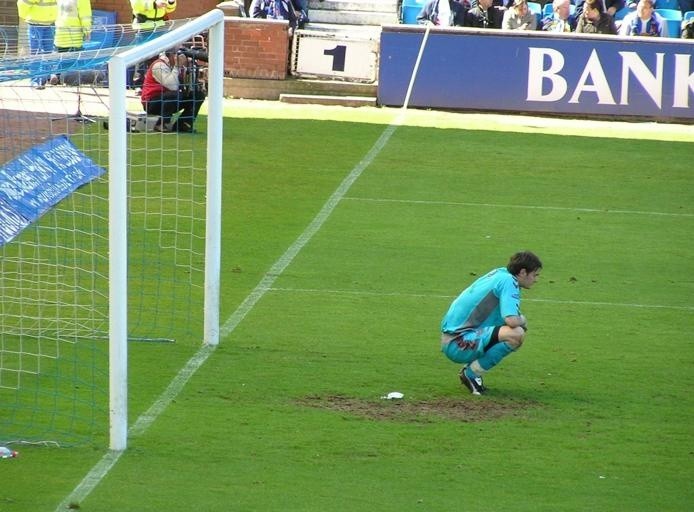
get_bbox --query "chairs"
[398,0,693,46]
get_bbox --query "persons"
[139,40,208,132]
[440,249,543,397]
[129,0,178,88]
[417,0,694,39]
[15,0,60,89]
[51,0,93,86]
[238,0,301,37]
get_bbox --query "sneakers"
[458,367,489,396]
[173,121,197,133]
[153,123,169,133]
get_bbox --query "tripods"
[52,72,97,123]
